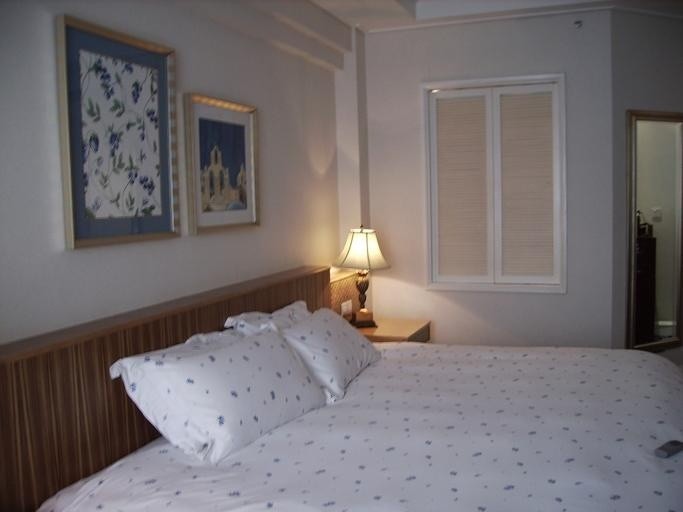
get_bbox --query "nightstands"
[354,314,432,347]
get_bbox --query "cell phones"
[654,440,683,458]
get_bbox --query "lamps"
[335,228,391,330]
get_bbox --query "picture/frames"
[182,94,260,236]
[56,12,177,250]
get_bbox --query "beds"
[0,265,683,512]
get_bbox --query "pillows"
[260,307,382,401]
[107,321,335,468]
[224,296,312,335]
